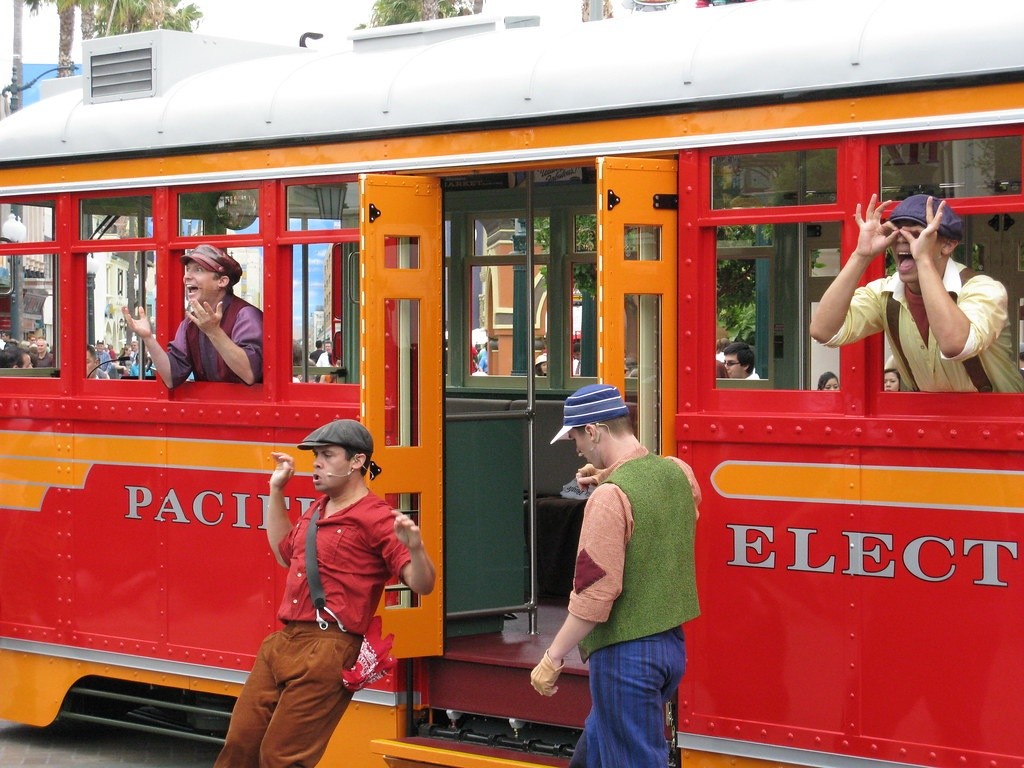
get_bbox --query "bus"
[0,1,1024,767]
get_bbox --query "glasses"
[723,360,741,367]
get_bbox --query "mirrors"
[214,188,260,230]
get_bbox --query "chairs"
[440,397,636,596]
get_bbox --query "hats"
[549,385,630,445]
[297,419,373,454]
[535,355,547,365]
[179,244,242,286]
[891,194,964,242]
[97,339,104,344]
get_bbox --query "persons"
[537,342,586,376]
[471,338,489,375]
[123,244,263,389]
[883,368,901,393]
[530,384,700,768]
[0,329,157,380]
[817,371,841,391]
[212,419,436,768]
[292,338,335,383]
[715,328,762,379]
[810,193,1024,394]
